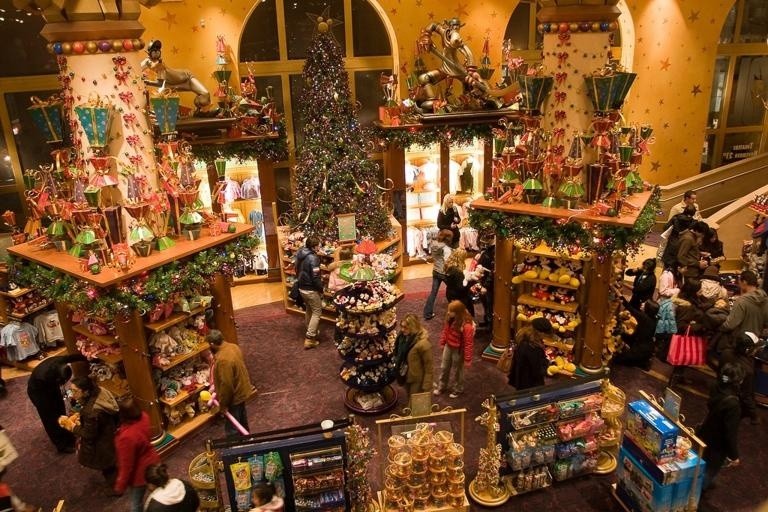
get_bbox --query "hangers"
[8,317,24,329]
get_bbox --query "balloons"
[200,390,249,436]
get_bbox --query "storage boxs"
[613,394,707,512]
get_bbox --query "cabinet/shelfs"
[405,136,492,268]
[190,156,270,287]
[0,276,54,320]
[199,412,362,511]
[460,181,655,381]
[330,237,400,416]
[493,377,627,502]
[5,215,258,465]
[738,205,767,266]
[271,196,402,324]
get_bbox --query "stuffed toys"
[330,254,401,383]
[511,251,585,375]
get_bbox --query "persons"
[507,318,554,391]
[418,19,505,111]
[392,313,433,413]
[423,194,495,397]
[140,40,211,109]
[328,247,353,346]
[206,329,258,437]
[251,479,285,512]
[294,235,325,348]
[26,355,201,512]
[619,191,768,512]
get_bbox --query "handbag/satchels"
[667,334,707,365]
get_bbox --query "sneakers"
[449,394,460,398]
[424,313,435,319]
[433,389,444,395]
[304,338,319,349]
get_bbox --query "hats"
[534,318,552,335]
